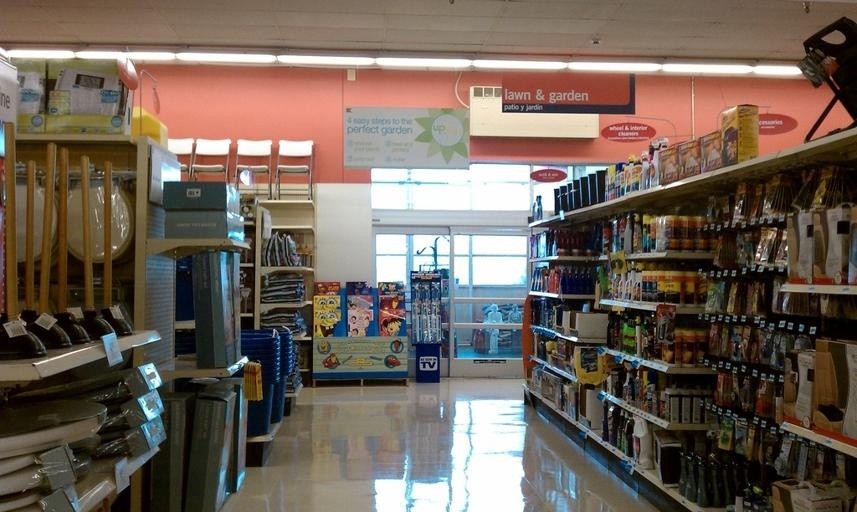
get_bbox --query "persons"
[488,305,502,354]
[509,304,522,347]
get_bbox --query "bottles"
[610,362,661,405]
[606,264,709,307]
[532,298,557,327]
[606,137,667,202]
[533,170,606,223]
[606,312,657,355]
[530,265,595,295]
[602,404,633,459]
[603,212,715,254]
[676,450,753,507]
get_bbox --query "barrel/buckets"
[241,328,293,436]
[632,414,656,470]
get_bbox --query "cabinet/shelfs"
[526,126,857,512]
[0,135,317,512]
